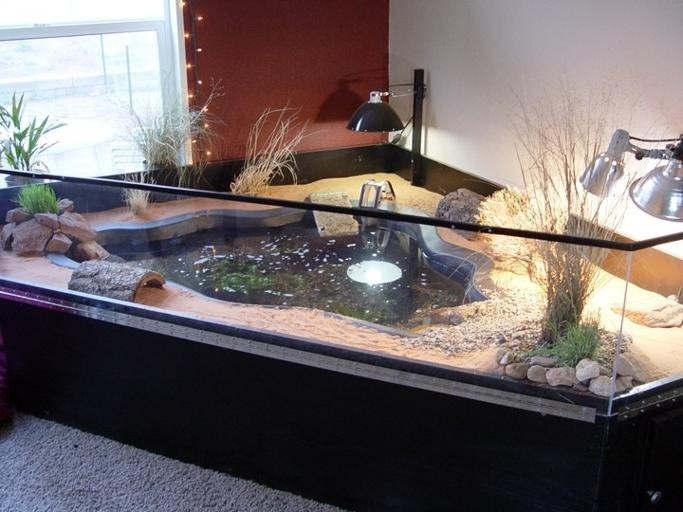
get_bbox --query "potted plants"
[111,76,229,187]
[2,90,68,185]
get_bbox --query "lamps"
[581,129,683,223]
[346,68,428,185]
